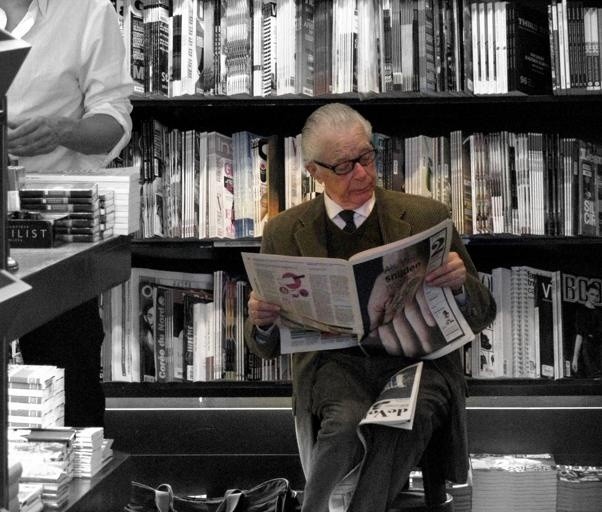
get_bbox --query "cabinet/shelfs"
[95,86,601,512]
[2,23,140,512]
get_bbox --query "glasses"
[313,142,378,175]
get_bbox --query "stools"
[386,419,456,510]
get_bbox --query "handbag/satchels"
[122,477,303,512]
